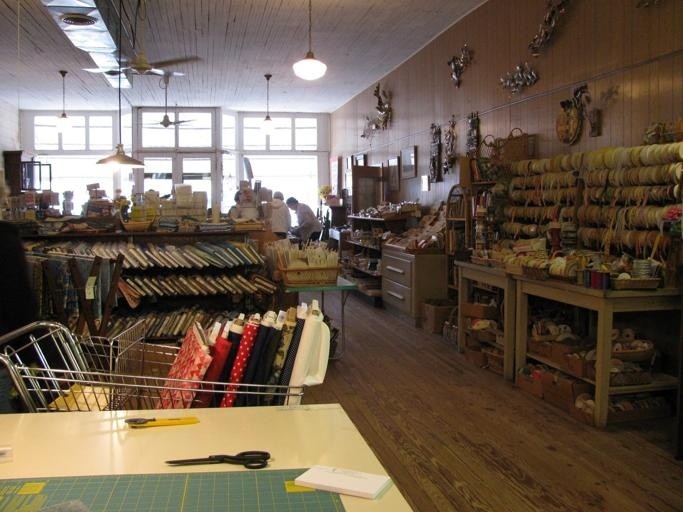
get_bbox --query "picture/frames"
[330,144,418,198]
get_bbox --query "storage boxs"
[421,297,452,332]
[459,303,503,372]
[519,330,588,416]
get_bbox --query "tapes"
[487,144,682,289]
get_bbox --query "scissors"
[164,451,270,469]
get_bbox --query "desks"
[512,272,682,430]
[328,229,351,271]
[273,274,359,354]
[454,257,523,382]
[0,402,415,512]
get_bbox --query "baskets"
[461,302,495,318]
[480,349,504,376]
[119,193,157,231]
[442,305,480,349]
[470,256,546,280]
[608,278,661,291]
[611,340,654,362]
[421,297,456,334]
[275,261,343,286]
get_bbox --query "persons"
[227,190,242,221]
[265,191,291,239]
[284,196,321,249]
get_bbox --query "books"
[20,238,320,408]
[293,463,392,499]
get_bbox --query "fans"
[138,73,195,129]
[81,1,203,78]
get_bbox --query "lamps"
[290,0,329,81]
[58,70,70,133]
[258,74,276,135]
[96,0,145,168]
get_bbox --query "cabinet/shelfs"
[471,158,500,303]
[0,221,285,363]
[1,151,53,196]
[381,247,450,329]
[336,205,418,306]
[342,164,381,225]
[445,183,476,335]
